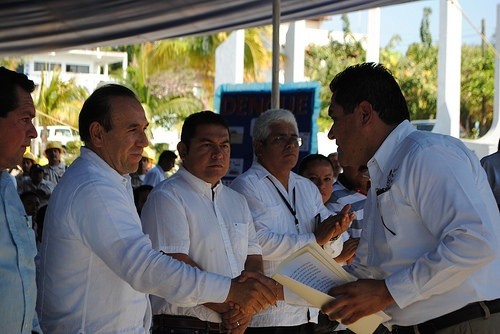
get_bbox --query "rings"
[236,321,240,325]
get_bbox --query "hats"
[22,152,37,164]
[43,141,63,157]
[30,164,44,171]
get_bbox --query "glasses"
[268,136,303,148]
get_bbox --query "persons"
[37,85,279,333]
[139,110,267,334]
[19,164,56,221]
[327,62,500,334]
[328,152,343,184]
[41,141,69,185]
[296,154,361,333]
[0,66,44,334]
[480,138,500,215]
[143,150,177,188]
[13,152,37,187]
[324,158,371,280]
[130,146,156,187]
[229,106,355,333]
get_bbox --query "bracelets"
[331,234,341,241]
